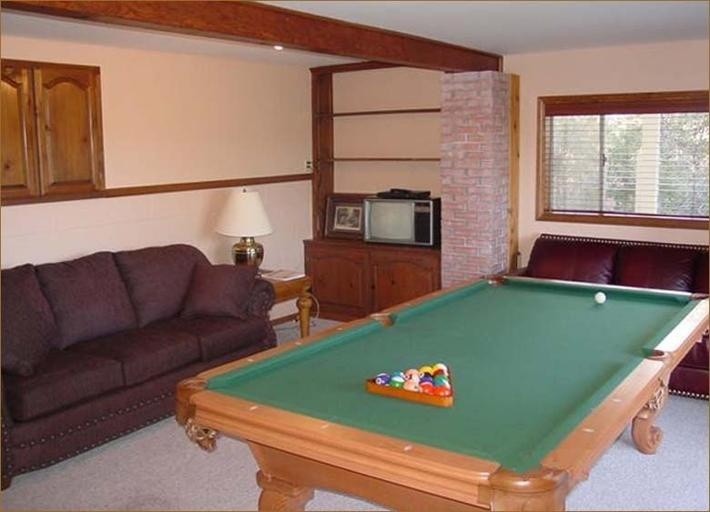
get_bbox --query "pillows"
[180,260,258,321]
[1,268,64,379]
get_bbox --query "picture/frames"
[324,194,364,240]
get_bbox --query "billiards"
[595,291,607,304]
[375,362,451,396]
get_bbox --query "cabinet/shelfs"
[0,56,107,206]
[301,238,441,323]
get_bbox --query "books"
[257,269,307,284]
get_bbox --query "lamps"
[214,189,274,269]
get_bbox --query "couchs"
[1,241,277,490]
[505,234,710,399]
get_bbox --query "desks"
[212,264,313,338]
[174,273,710,511]
